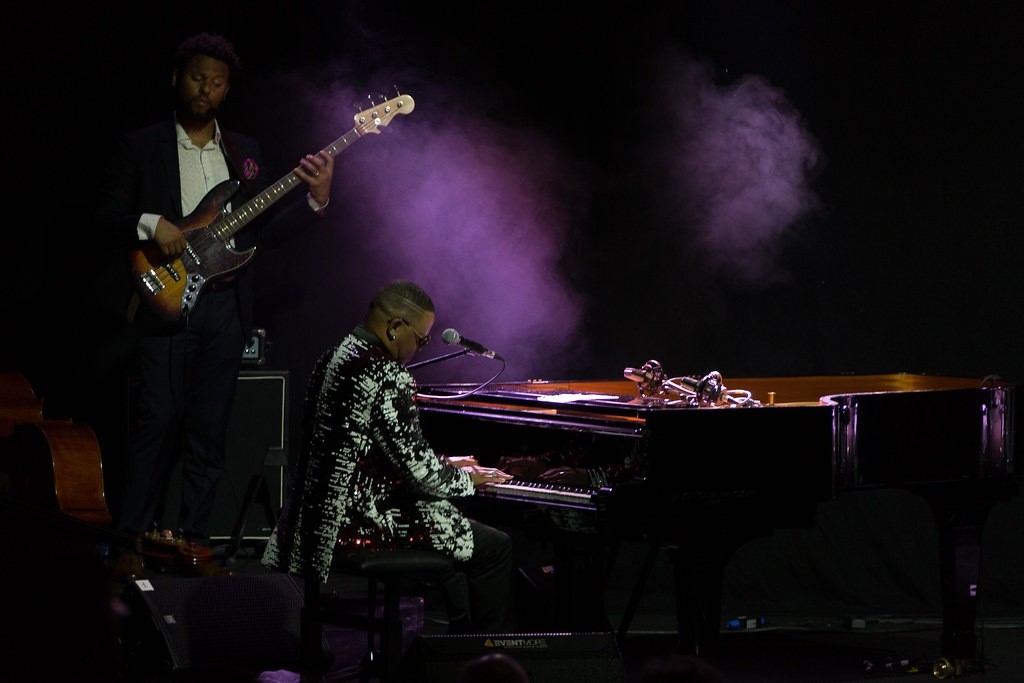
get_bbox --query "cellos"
[1,364,217,569]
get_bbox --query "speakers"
[206,368,294,545]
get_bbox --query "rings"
[315,172,319,176]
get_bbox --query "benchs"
[302,547,448,683]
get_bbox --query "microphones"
[442,329,506,362]
[681,375,741,404]
[623,368,694,397]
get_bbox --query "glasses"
[388,318,428,348]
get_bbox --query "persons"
[101,34,334,580]
[260,281,530,636]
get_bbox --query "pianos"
[411,367,1022,683]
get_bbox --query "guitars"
[123,80,419,329]
